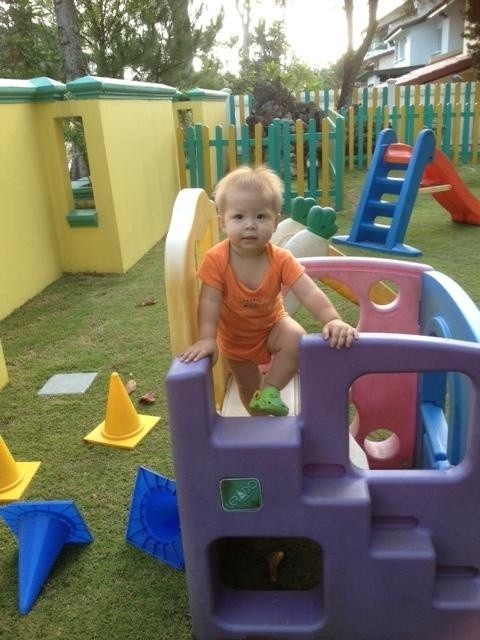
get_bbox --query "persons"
[180,164,359,417]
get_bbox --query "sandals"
[249,386,290,417]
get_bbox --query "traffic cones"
[1,499,95,615]
[86,371,158,449]
[125,466,183,568]
[1,432,42,498]
